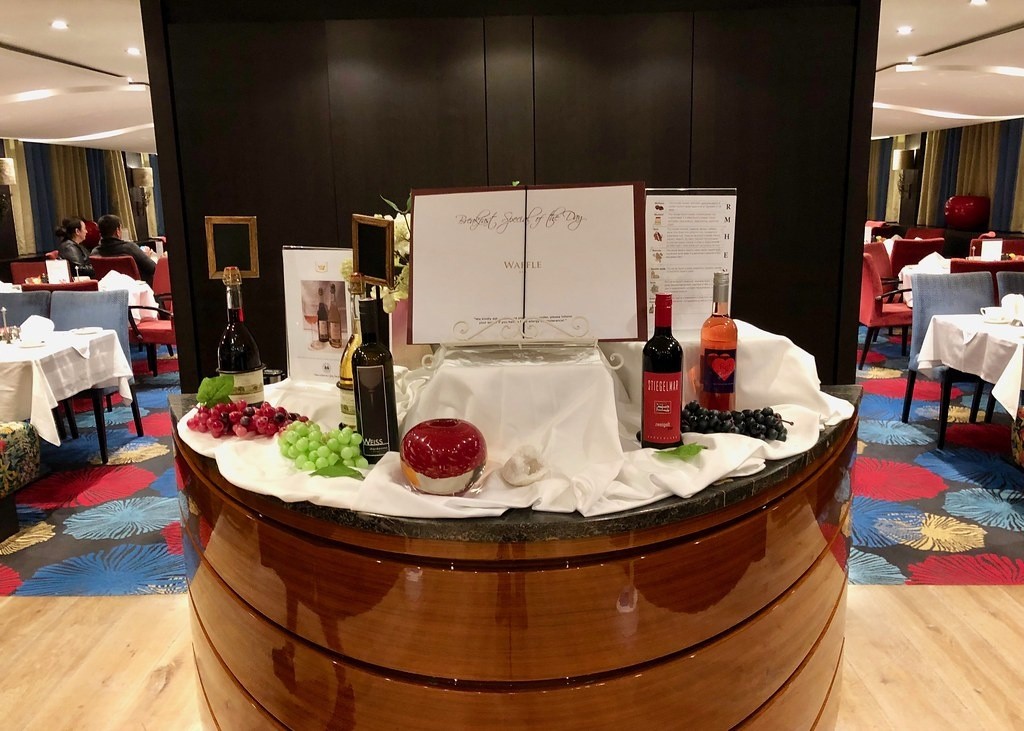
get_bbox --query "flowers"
[341,181,520,313]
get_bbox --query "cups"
[981,306,1004,320]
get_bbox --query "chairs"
[859,221,1024,449]
[10,236,177,465]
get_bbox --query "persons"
[55,215,95,278]
[91,214,167,319]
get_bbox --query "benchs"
[0,422,40,543]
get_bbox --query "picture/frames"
[204,216,259,279]
[352,213,395,288]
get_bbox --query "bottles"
[337,275,366,431]
[317,288,329,342]
[641,293,684,449]
[698,272,738,410]
[350,297,401,464]
[218,267,264,409]
[329,284,342,348]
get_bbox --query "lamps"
[0,158,16,222]
[132,167,154,216]
[893,149,914,200]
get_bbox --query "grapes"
[637,400,787,442]
[186,400,368,472]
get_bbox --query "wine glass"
[302,297,319,350]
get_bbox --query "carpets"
[0,325,1023,598]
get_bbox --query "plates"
[72,326,103,334]
[984,320,1008,323]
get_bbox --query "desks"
[898,259,950,307]
[917,314,1024,423]
[170,384,863,730]
[0,330,133,448]
[97,282,160,326]
